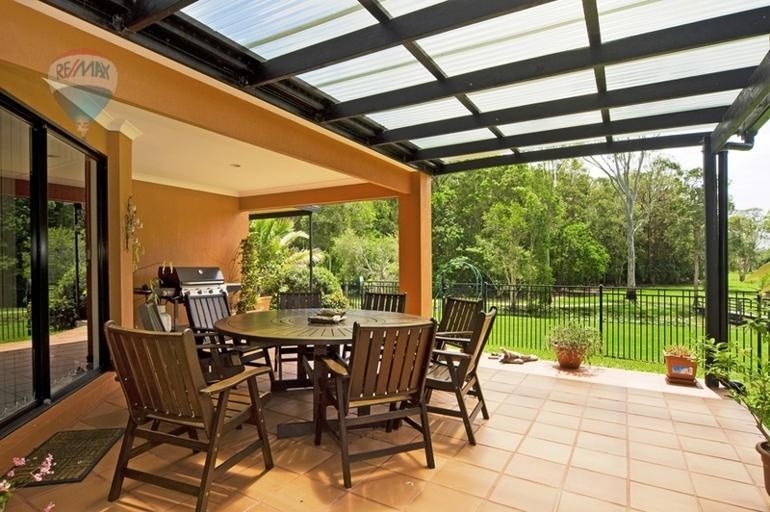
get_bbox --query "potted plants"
[146,278,172,332]
[547,322,600,369]
[691,295,770,495]
[663,345,699,386]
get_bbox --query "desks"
[213,308,428,445]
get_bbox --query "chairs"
[395,306,498,446]
[184,292,275,428]
[104,320,273,512]
[341,292,406,361]
[314,317,437,489]
[426,296,483,403]
[273,291,322,381]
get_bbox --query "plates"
[308,310,346,323]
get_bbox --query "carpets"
[0,427,127,488]
